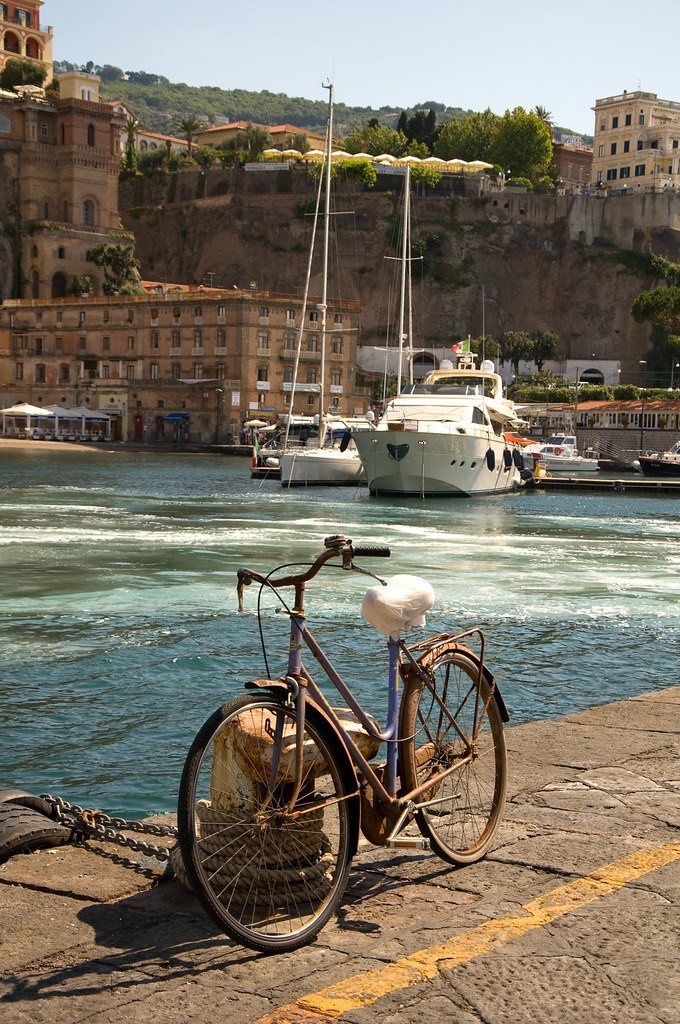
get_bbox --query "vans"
[569,382,589,393]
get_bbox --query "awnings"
[163,413,189,421]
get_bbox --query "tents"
[0,403,111,437]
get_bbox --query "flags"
[451,334,472,354]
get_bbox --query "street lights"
[639,361,646,455]
[671,356,679,388]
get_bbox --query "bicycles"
[177,533,509,952]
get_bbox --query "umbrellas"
[263,149,493,172]
[244,419,285,432]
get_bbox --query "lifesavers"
[555,448,561,456]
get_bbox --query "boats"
[638,441,680,477]
[350,285,598,499]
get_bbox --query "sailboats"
[244,53,424,488]
